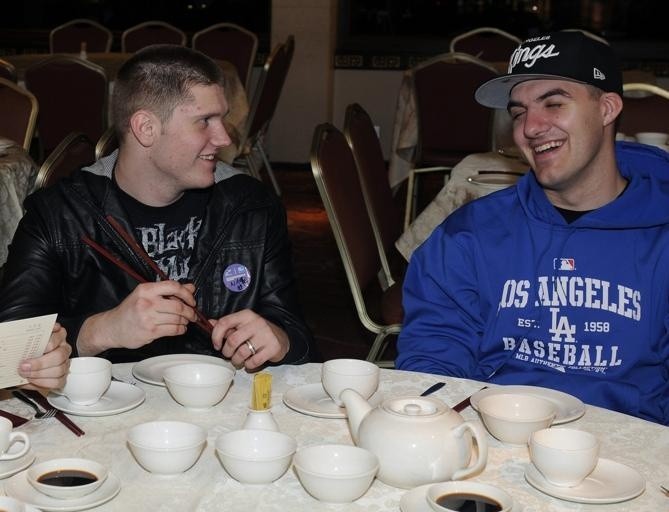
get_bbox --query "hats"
[474,28,623,110]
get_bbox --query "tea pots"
[338,388,489,487]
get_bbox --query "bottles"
[238,405,280,430]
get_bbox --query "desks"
[0,356,669,511]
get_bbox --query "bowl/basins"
[294,443,378,500]
[479,391,557,443]
[128,418,209,472]
[217,428,295,484]
[164,363,233,409]
[28,457,108,499]
[425,479,512,511]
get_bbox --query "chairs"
[293,20,668,360]
[0,10,300,188]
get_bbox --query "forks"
[12,389,57,420]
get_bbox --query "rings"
[246,338,256,357]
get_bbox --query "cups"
[321,360,381,403]
[528,427,600,484]
[53,358,112,405]
[0,414,30,460]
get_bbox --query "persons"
[394,26,669,427]
[14,321,73,392]
[0,43,318,370]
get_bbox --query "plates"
[7,464,120,510]
[523,460,646,504]
[469,384,584,424]
[284,383,384,417]
[44,383,147,417]
[132,350,234,386]
[1,442,34,480]
[398,483,540,512]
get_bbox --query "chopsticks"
[80,212,215,337]
[25,386,84,437]
